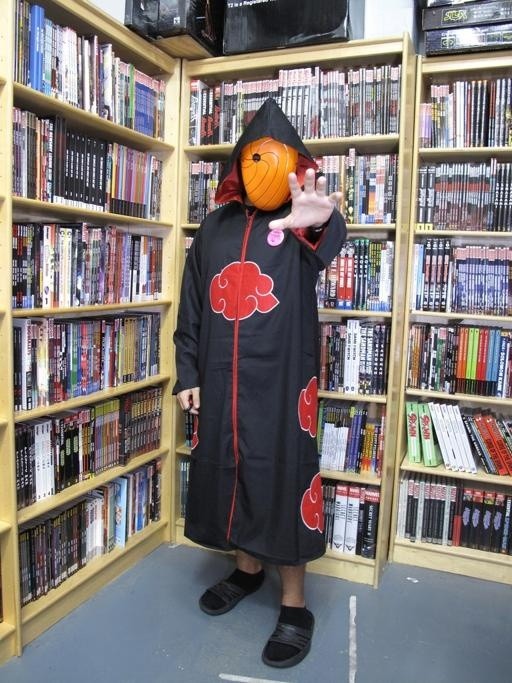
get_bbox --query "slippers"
[261,609,313,667]
[199,579,247,615]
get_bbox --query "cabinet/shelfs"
[182,40,408,594]
[389,62,510,586]
[10,1,181,657]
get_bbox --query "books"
[318,317,512,400]
[13,384,161,608]
[418,78,512,147]
[178,408,193,519]
[13,220,163,413]
[314,398,387,559]
[310,147,512,233]
[183,160,223,263]
[396,394,512,556]
[189,65,403,147]
[315,234,512,317]
[11,0,169,221]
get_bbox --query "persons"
[172,93,349,673]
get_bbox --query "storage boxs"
[418,4,512,58]
[213,1,369,57]
[124,1,212,50]
